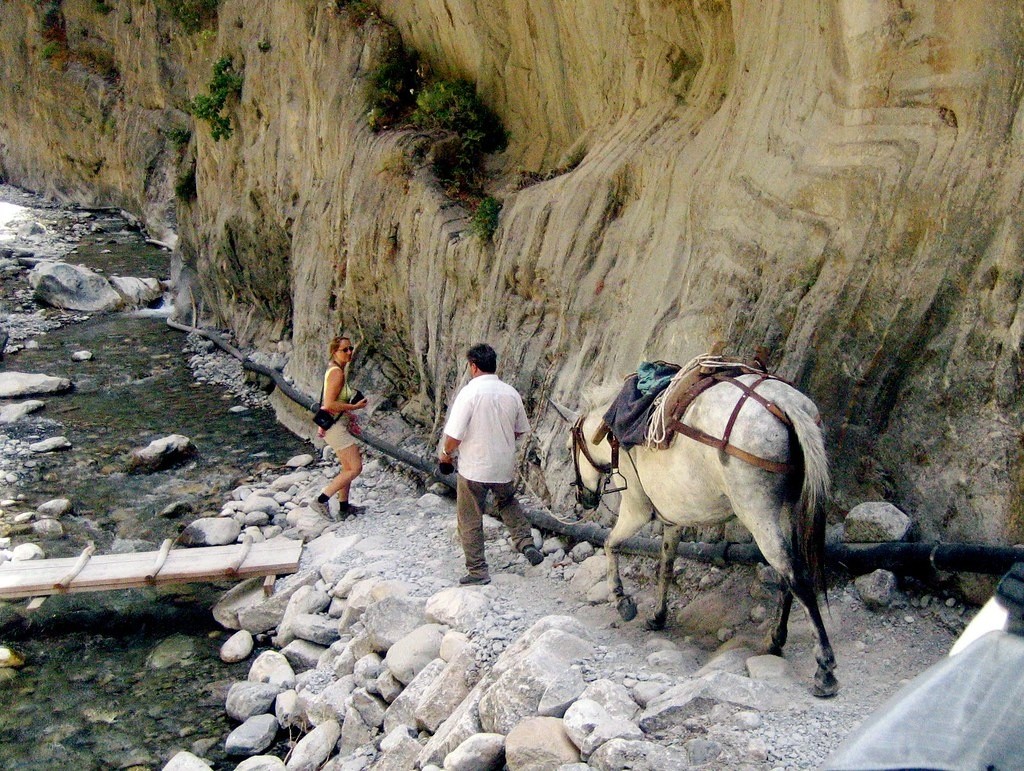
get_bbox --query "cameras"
[350,391,367,405]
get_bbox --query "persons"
[439,343,544,586]
[310,336,368,523]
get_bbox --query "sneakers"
[460,567,491,586]
[337,504,366,520]
[309,496,335,522]
[523,545,544,566]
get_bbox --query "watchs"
[443,447,456,457]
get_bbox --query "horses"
[544,353,839,699]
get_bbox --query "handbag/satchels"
[312,407,335,431]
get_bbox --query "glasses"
[338,347,353,352]
